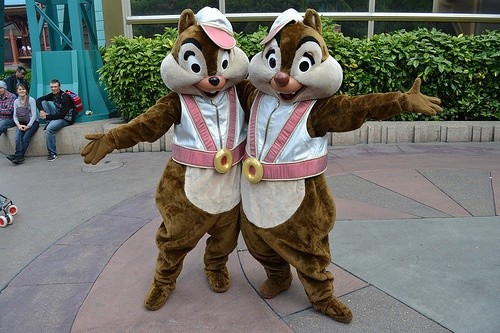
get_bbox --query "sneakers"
[47,152,58,161]
[43,126,47,130]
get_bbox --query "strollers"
[0,194,19,228]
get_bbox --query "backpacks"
[61,90,83,111]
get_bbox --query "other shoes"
[7,155,19,165]
[12,158,25,163]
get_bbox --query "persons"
[4,66,30,96]
[36,79,76,161]
[0,81,17,136]
[7,83,40,164]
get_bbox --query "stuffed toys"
[241,8,443,323]
[81,7,250,309]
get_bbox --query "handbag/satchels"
[16,95,30,124]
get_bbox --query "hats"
[195,6,236,50]
[0,81,7,89]
[259,8,304,45]
